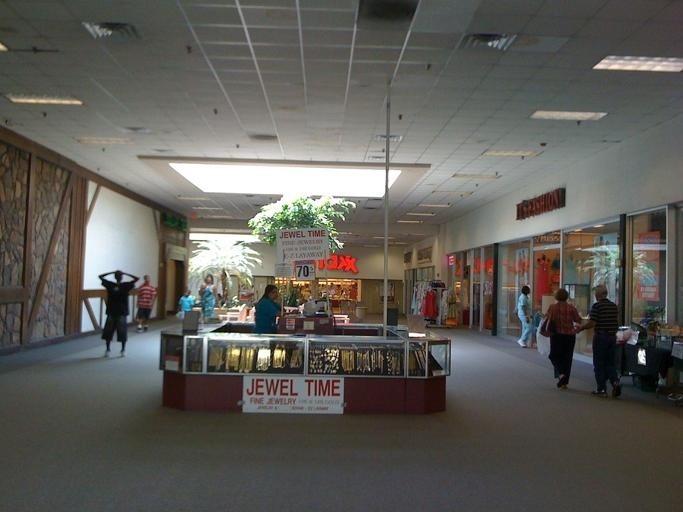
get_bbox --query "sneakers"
[592,382,622,398]
[517,339,526,347]
[137,326,148,332]
[556,375,569,389]
[104,351,126,357]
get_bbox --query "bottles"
[655,329,672,351]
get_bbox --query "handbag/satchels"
[540,316,550,336]
[176,311,185,320]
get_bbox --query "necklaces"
[341,349,402,375]
[225,346,303,374]
[410,350,425,371]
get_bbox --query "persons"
[134,274,157,335]
[514,286,532,347]
[179,287,197,317]
[98,270,139,358]
[326,283,335,296]
[252,285,282,335]
[576,286,622,398]
[544,289,581,390]
[198,273,219,325]
[299,283,310,304]
